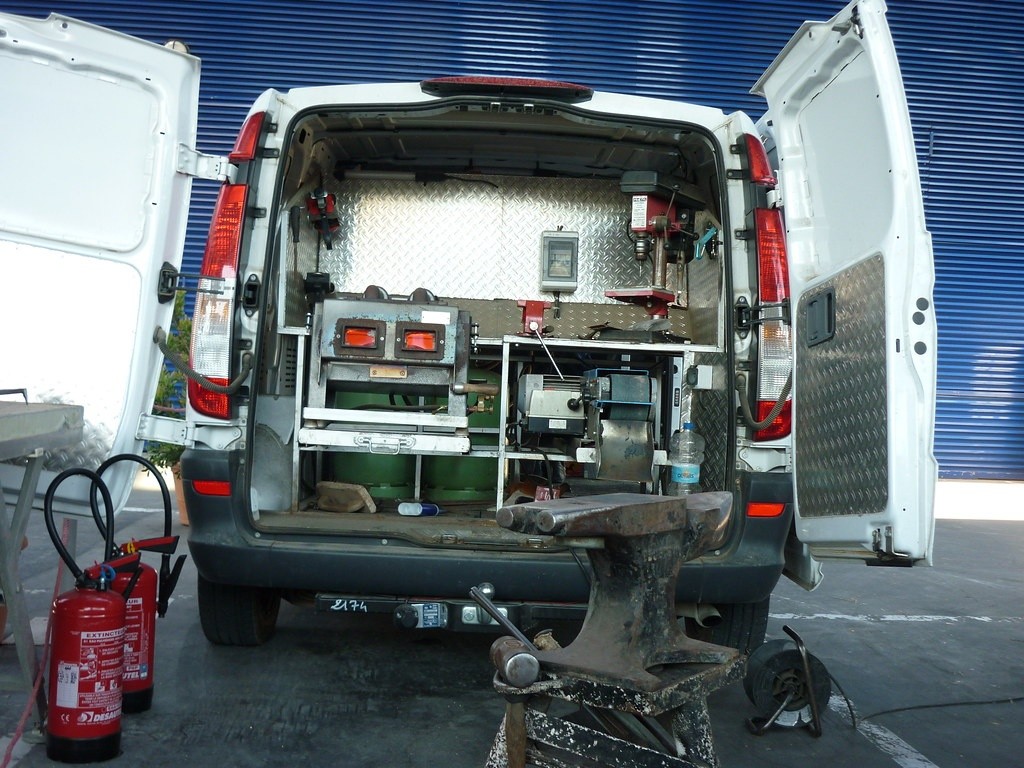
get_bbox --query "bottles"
[667,424,705,499]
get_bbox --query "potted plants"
[141,279,195,525]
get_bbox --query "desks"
[0,400,85,733]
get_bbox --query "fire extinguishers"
[45,452,188,766]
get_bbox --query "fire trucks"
[0,1,939,669]
